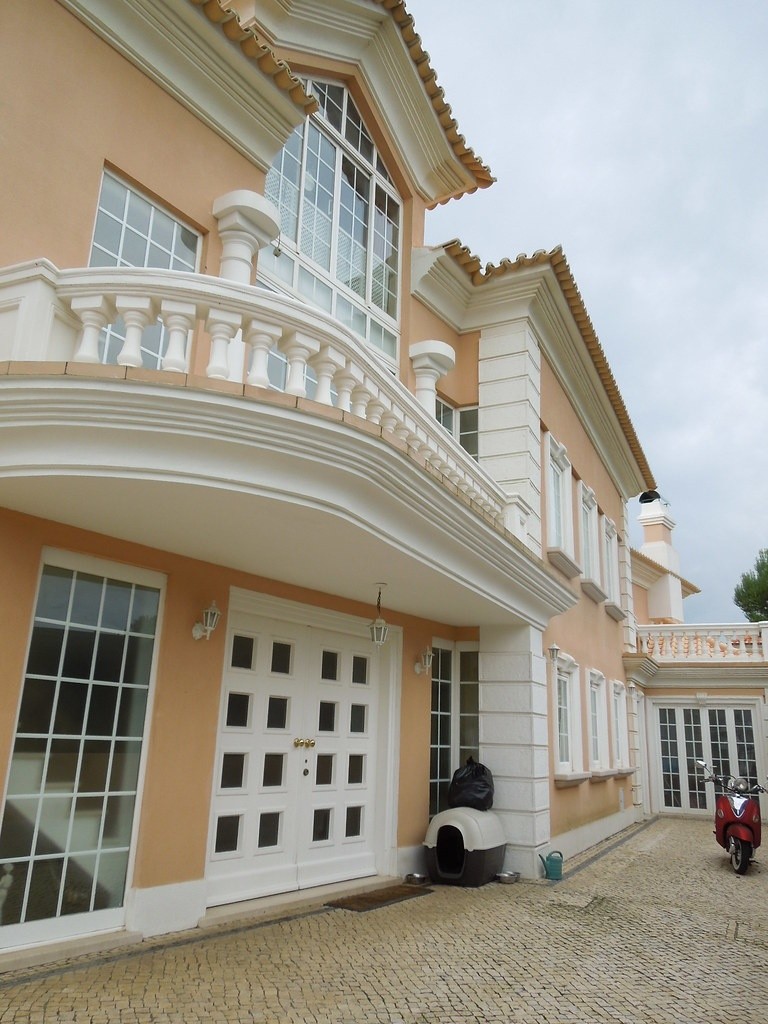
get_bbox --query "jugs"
[539,850,564,880]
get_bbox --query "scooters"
[694,760,768,876]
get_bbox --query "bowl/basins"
[405,874,426,884]
[496,870,521,884]
